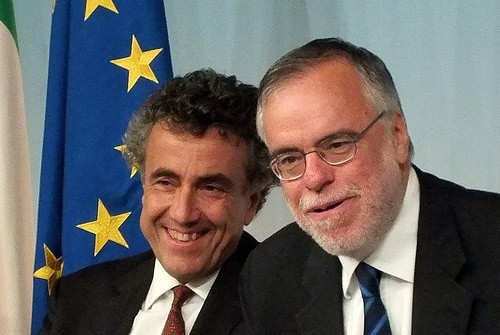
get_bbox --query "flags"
[30,0,173,335]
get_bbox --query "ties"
[355,261,392,335]
[161,285,195,335]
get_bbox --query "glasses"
[267,111,384,181]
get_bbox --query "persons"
[37,68,281,335]
[237,37,500,335]
